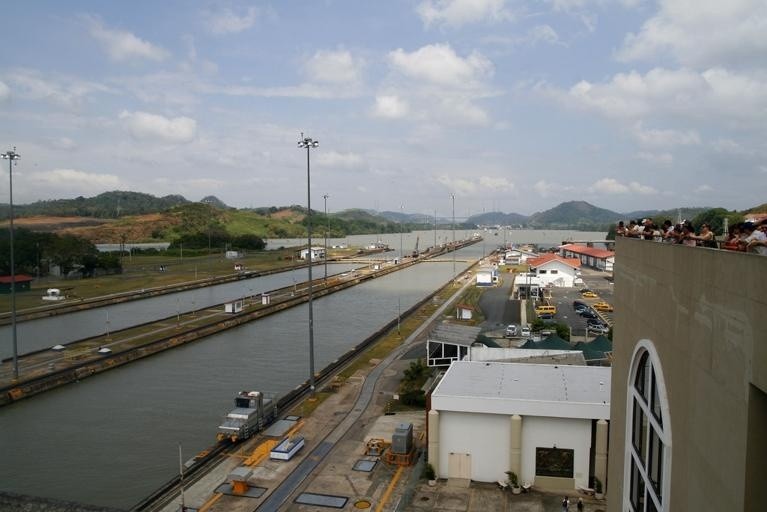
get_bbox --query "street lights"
[295,134,323,396]
[322,192,330,285]
[396,191,505,279]
[0,146,21,381]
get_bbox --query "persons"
[616,217,767,255]
[562,495,570,511]
[576,497,583,511]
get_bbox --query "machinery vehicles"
[215,389,282,443]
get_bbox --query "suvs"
[506,324,518,337]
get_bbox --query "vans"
[534,305,557,316]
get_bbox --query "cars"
[573,288,615,334]
[537,312,554,320]
[520,327,531,337]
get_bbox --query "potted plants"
[503,469,523,494]
[592,476,604,501]
[422,461,437,487]
